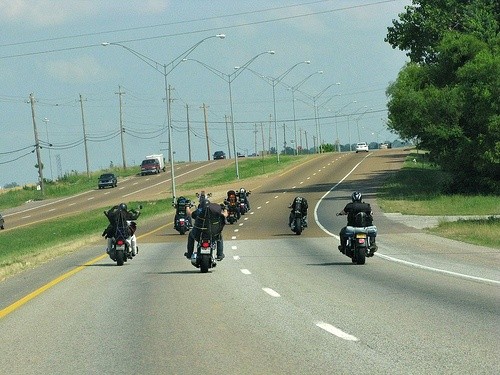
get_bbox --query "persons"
[183,199,225,262]
[172,187,250,233]
[103,203,138,256]
[336,191,378,256]
[287,197,309,230]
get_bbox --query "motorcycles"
[172,195,196,235]
[336,212,376,264]
[103,205,143,265]
[192,188,252,273]
[290,210,307,235]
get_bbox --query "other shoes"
[338,245,345,254]
[184,252,192,258]
[372,245,378,252]
[217,254,225,261]
[106,247,112,254]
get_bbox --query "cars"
[212,151,226,160]
[98,174,117,189]
[379,143,388,149]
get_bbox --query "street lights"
[180,50,276,179]
[42,117,53,181]
[262,70,369,156]
[100,34,226,204]
[233,60,311,162]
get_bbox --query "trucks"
[139,154,166,176]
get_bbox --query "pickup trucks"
[355,142,368,153]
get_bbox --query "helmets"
[352,191,362,203]
[295,197,303,203]
[119,203,127,211]
[200,199,211,206]
